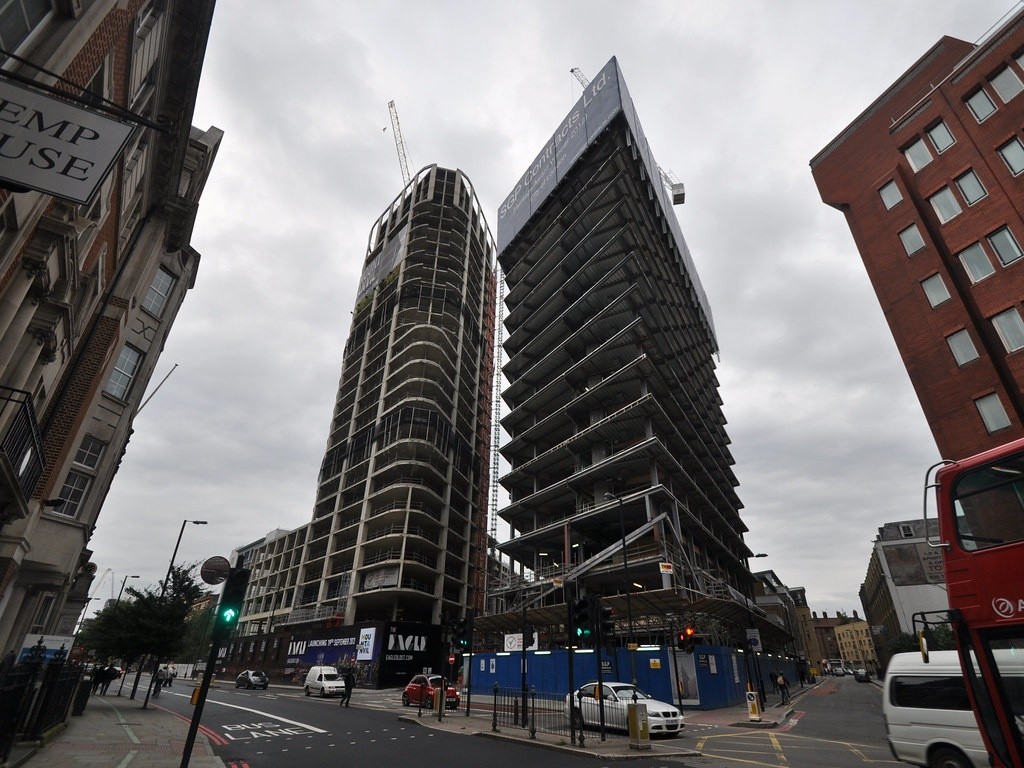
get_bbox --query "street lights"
[69,597,101,659]
[111,574,140,618]
[130,519,208,700]
[846,629,869,674]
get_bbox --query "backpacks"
[777,675,784,685]
[108,668,117,681]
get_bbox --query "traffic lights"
[575,600,590,640]
[457,619,466,640]
[603,607,616,641]
[678,632,684,649]
[684,627,694,654]
[216,568,251,631]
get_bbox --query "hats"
[779,671,783,674]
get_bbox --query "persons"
[830,668,834,676]
[339,668,356,708]
[91,663,118,696]
[776,670,790,705]
[151,666,177,699]
[769,668,779,695]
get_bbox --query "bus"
[911,437,1024,768]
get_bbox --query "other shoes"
[90,692,107,696]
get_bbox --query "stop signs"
[449,653,455,665]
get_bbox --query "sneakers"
[340,704,342,707]
[345,704,349,707]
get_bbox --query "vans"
[304,665,346,697]
[883,649,1024,768]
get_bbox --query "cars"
[855,669,870,682]
[401,674,460,709]
[235,670,270,690]
[84,663,123,679]
[563,682,686,737]
[831,668,853,677]
